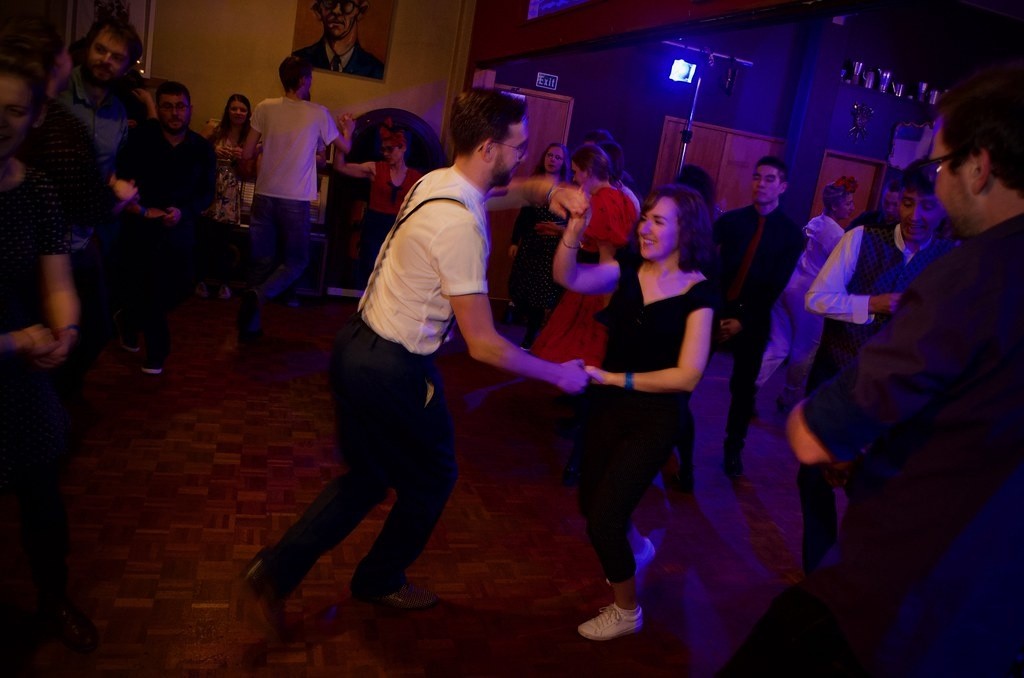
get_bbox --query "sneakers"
[577,602,643,642]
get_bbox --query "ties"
[727,216,767,301]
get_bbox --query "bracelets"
[546,187,564,203]
[563,234,582,251]
[624,370,633,391]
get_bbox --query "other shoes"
[140,352,166,374]
[237,327,264,345]
[723,451,743,476]
[40,596,98,653]
[674,464,694,495]
[235,289,257,330]
[116,309,139,352]
[239,554,286,632]
[219,281,231,299]
[196,280,208,298]
[374,582,438,611]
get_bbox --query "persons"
[672,166,724,221]
[529,188,637,489]
[331,110,424,299]
[749,176,855,410]
[235,55,355,344]
[236,87,592,641]
[200,93,264,300]
[845,164,905,231]
[0,9,217,655]
[552,184,728,642]
[797,156,957,578]
[510,128,642,353]
[710,155,810,478]
[710,55,1024,678]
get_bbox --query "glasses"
[920,146,970,183]
[478,137,528,161]
[160,103,195,112]
